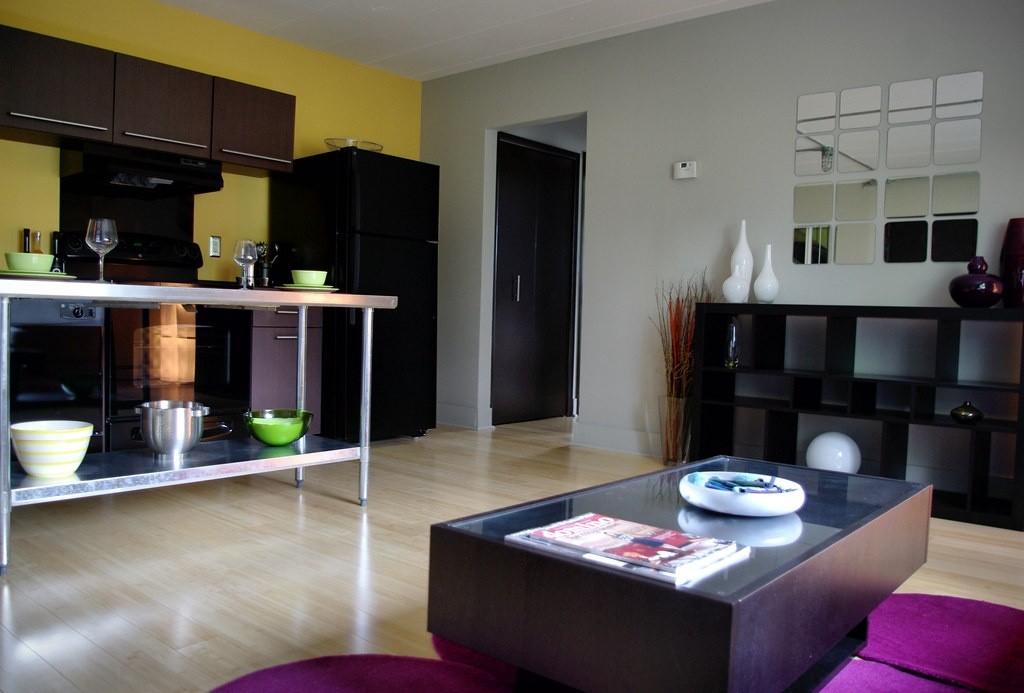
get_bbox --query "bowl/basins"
[324,138,383,153]
[243,409,313,448]
[9,420,94,478]
[678,505,802,547]
[291,270,328,286]
[4,252,55,272]
[680,472,805,517]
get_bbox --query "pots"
[135,400,210,454]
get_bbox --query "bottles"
[32,231,43,254]
[754,244,779,304]
[729,220,754,303]
[23,228,30,253]
[51,231,61,273]
[948,256,1004,308]
[951,401,984,426]
[722,265,750,302]
[725,315,742,369]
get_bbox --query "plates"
[274,286,339,293]
[283,284,333,288]
[0,270,78,280]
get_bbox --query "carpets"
[215,594,1024,693]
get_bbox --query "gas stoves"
[59,228,244,289]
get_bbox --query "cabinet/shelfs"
[0,23,296,177]
[689,299,1024,534]
[252,307,322,438]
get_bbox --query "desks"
[0,282,404,575]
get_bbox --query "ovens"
[109,303,251,453]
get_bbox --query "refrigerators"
[267,145,441,446]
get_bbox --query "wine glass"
[233,240,258,290]
[85,218,118,283]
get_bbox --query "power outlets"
[210,235,220,257]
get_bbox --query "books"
[503,509,751,585]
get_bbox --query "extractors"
[60,147,223,203]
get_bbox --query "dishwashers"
[8,298,105,466]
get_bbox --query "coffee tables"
[429,455,935,693]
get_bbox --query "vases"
[725,322,740,369]
[949,219,1024,309]
[724,219,779,302]
[657,396,687,468]
